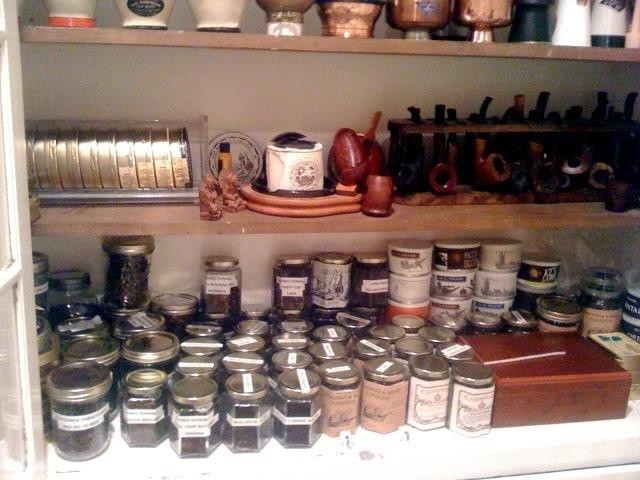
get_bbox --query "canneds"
[516,253,640,404]
[33,235,540,462]
[265,142,323,194]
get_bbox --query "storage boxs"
[454,330,633,433]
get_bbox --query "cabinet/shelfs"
[0,0,640,478]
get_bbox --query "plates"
[206,132,265,187]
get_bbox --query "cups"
[590,0,630,47]
[317,0,384,37]
[551,0,592,46]
[185,1,248,32]
[507,1,554,44]
[386,1,452,40]
[362,175,395,217]
[453,1,513,41]
[112,0,177,29]
[46,1,98,28]
[255,1,315,36]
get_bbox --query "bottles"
[217,139,233,180]
[46,270,100,330]
